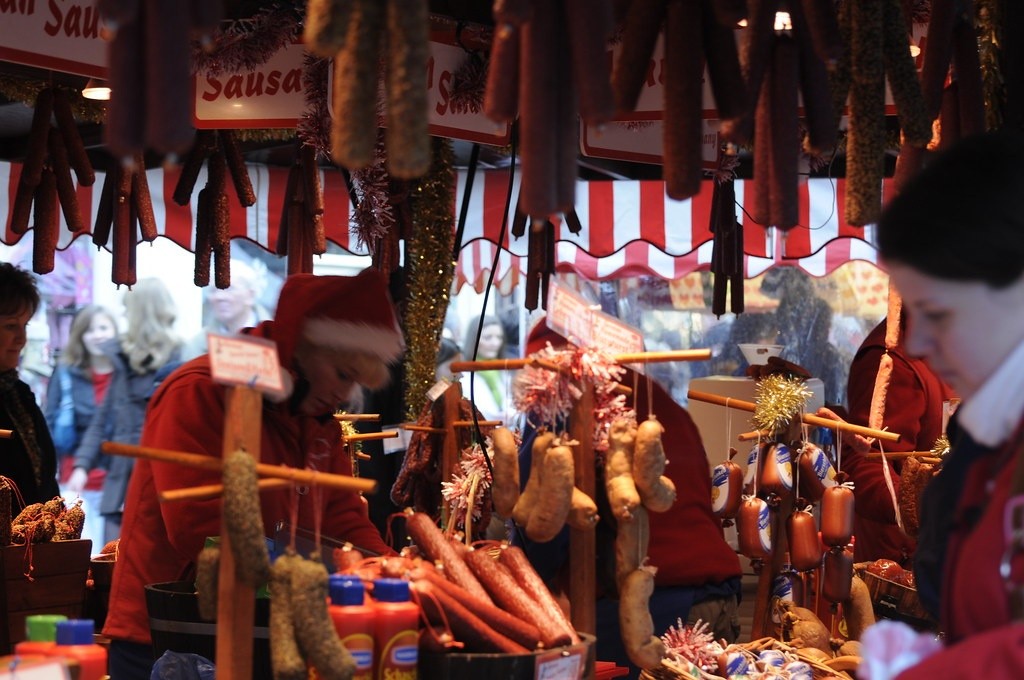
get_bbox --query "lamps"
[81,77,112,101]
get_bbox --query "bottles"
[327,582,373,680]
[308,575,360,680]
[46,619,107,680]
[372,578,419,680]
[16,615,68,657]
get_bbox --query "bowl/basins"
[738,344,784,366]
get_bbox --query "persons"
[100,266,405,680]
[854,129,1024,680]
[45,261,959,636]
[0,262,61,523]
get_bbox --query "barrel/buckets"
[90,552,116,620]
[417,632,597,680]
[144,581,273,680]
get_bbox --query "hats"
[267,265,405,403]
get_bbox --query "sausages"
[712,442,935,680]
[9,0,975,347]
[197,396,675,680]
[0,476,86,545]
[867,354,893,442]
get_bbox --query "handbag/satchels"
[53,366,77,448]
